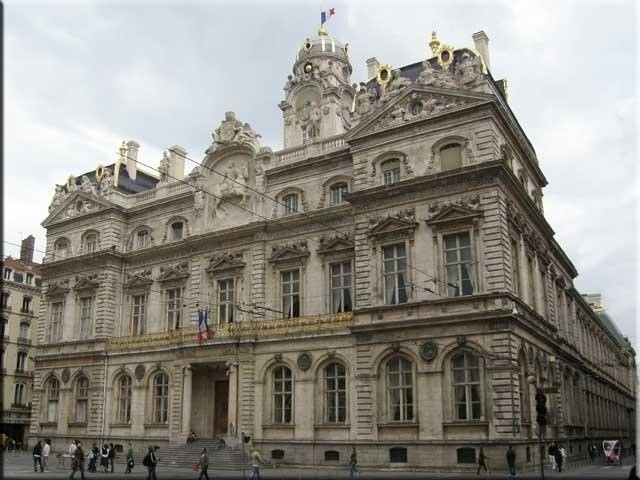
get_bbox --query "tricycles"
[602,439,623,465]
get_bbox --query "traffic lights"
[534,392,547,428]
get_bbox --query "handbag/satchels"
[71,461,78,469]
[192,465,199,471]
[129,458,134,467]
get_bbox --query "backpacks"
[143,455,148,466]
[113,448,117,457]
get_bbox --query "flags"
[196,307,211,346]
[321,7,336,25]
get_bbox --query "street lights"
[525,374,548,480]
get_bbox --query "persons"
[123,442,134,473]
[186,432,196,444]
[146,445,161,480]
[194,447,210,480]
[47,167,114,218]
[64,438,116,480]
[214,437,225,450]
[545,438,568,475]
[157,150,171,182]
[505,446,517,476]
[348,447,361,480]
[353,49,478,126]
[33,439,44,472]
[475,446,490,476]
[41,438,51,472]
[247,446,266,480]
[298,100,321,133]
[205,111,265,214]
